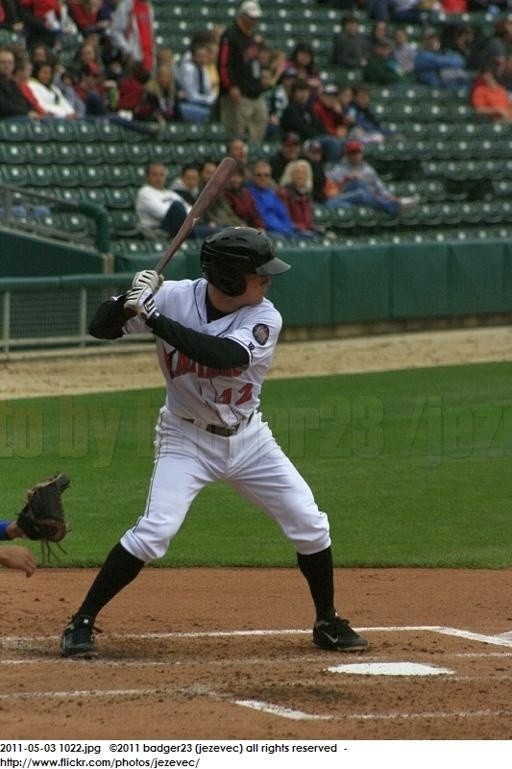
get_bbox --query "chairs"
[0,0,512,262]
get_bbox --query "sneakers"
[59,615,98,658]
[312,612,368,652]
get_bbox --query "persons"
[59,226,372,659]
[1,0,510,245]
[0,471,72,577]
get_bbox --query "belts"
[183,413,253,436]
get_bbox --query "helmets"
[200,227,292,297]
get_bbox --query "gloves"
[123,269,165,321]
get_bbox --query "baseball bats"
[124,158,235,319]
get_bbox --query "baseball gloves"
[17,472,72,542]
